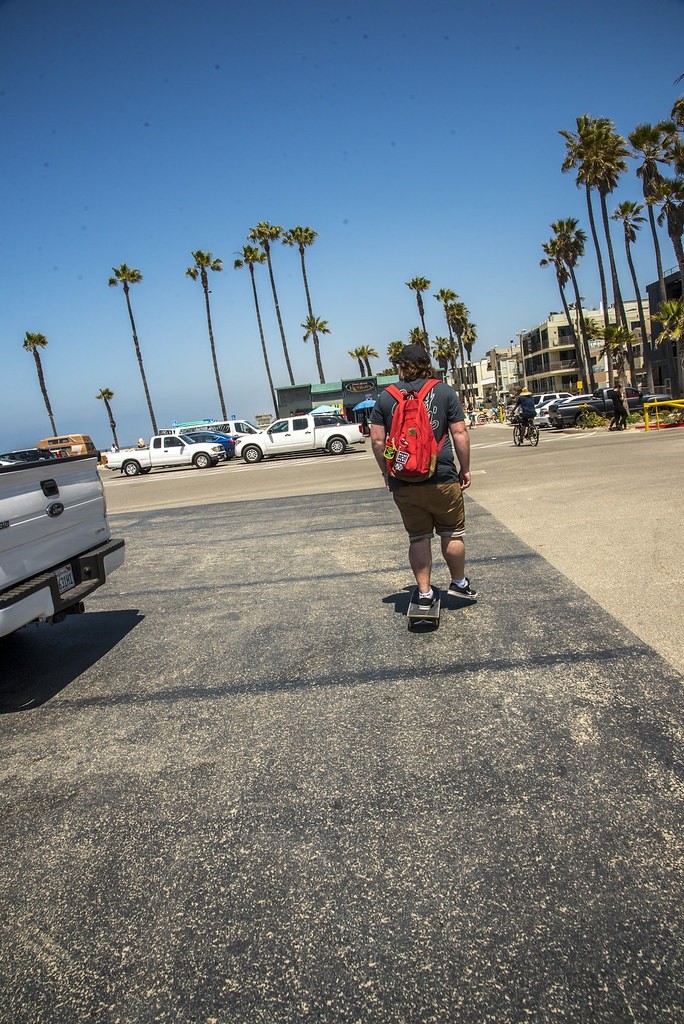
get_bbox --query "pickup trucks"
[549,386,673,429]
[0,451,125,638]
[235,416,364,463]
[105,434,227,477]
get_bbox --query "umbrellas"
[352,399,378,422]
[306,405,342,414]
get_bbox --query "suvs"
[0,448,55,460]
[185,431,237,462]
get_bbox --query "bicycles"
[511,411,539,447]
[477,412,504,424]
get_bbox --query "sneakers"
[447,578,477,600]
[419,586,437,610]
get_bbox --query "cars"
[532,394,594,427]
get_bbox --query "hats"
[392,345,431,365]
[519,388,532,396]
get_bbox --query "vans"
[176,420,263,437]
[532,392,573,408]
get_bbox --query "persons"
[608,388,630,431]
[370,342,479,612]
[486,397,492,404]
[612,384,629,431]
[510,387,537,448]
[110,443,119,453]
[360,411,371,437]
[466,402,477,430]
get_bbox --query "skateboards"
[407,584,441,631]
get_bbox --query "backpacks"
[384,379,442,482]
